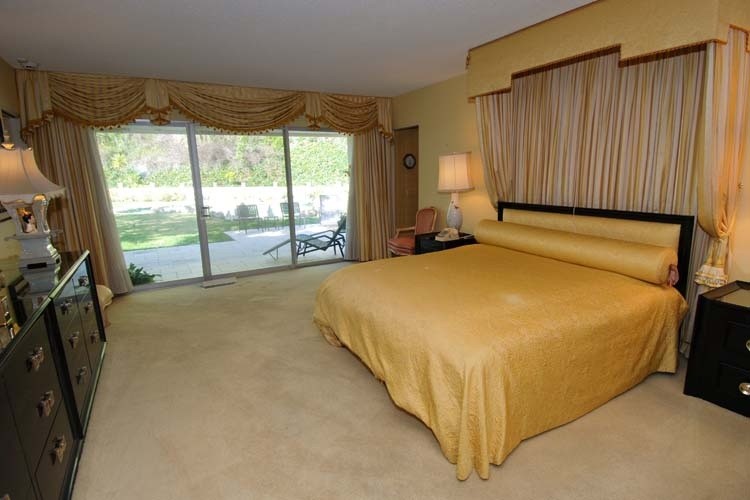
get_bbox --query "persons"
[23,211,37,233]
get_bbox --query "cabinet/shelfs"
[1,248,109,499]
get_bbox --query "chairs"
[237,205,263,234]
[278,200,305,229]
[386,205,439,256]
[264,215,345,259]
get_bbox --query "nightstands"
[682,279,750,420]
[412,230,477,254]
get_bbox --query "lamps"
[436,152,473,233]
[0,139,69,270]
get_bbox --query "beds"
[312,201,696,484]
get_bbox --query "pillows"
[474,218,679,293]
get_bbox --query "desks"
[263,215,280,231]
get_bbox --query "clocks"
[402,153,417,170]
